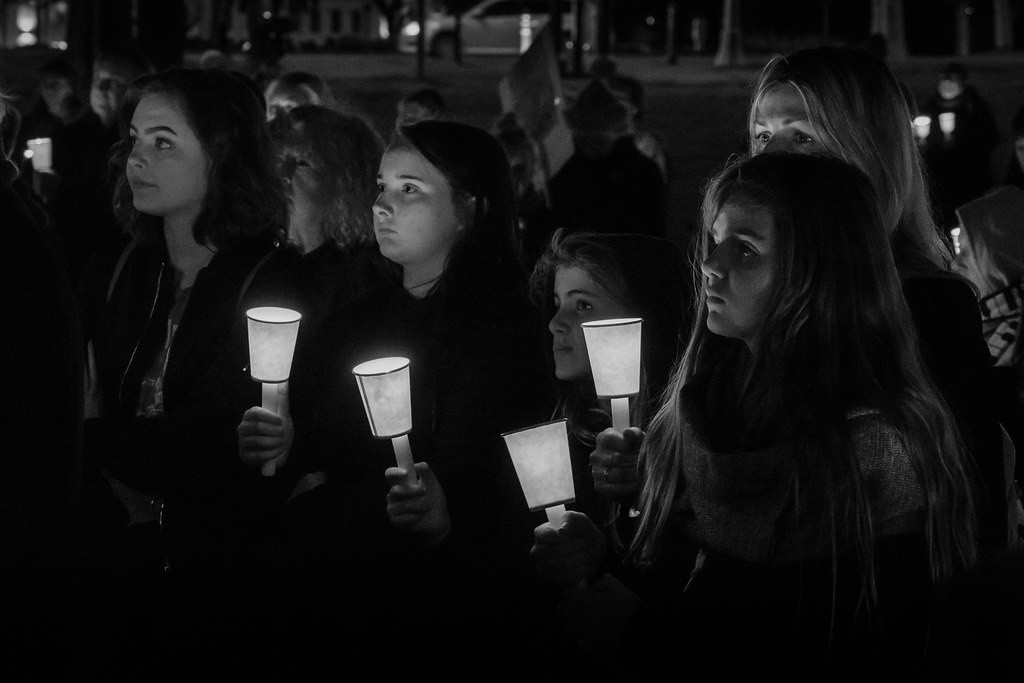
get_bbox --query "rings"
[603,466,612,483]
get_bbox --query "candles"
[582,318,642,430]
[502,417,575,528]
[354,357,417,487]
[245,307,302,477]
[26,139,52,173]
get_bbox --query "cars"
[397,0,577,62]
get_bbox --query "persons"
[2,41,1024,682]
